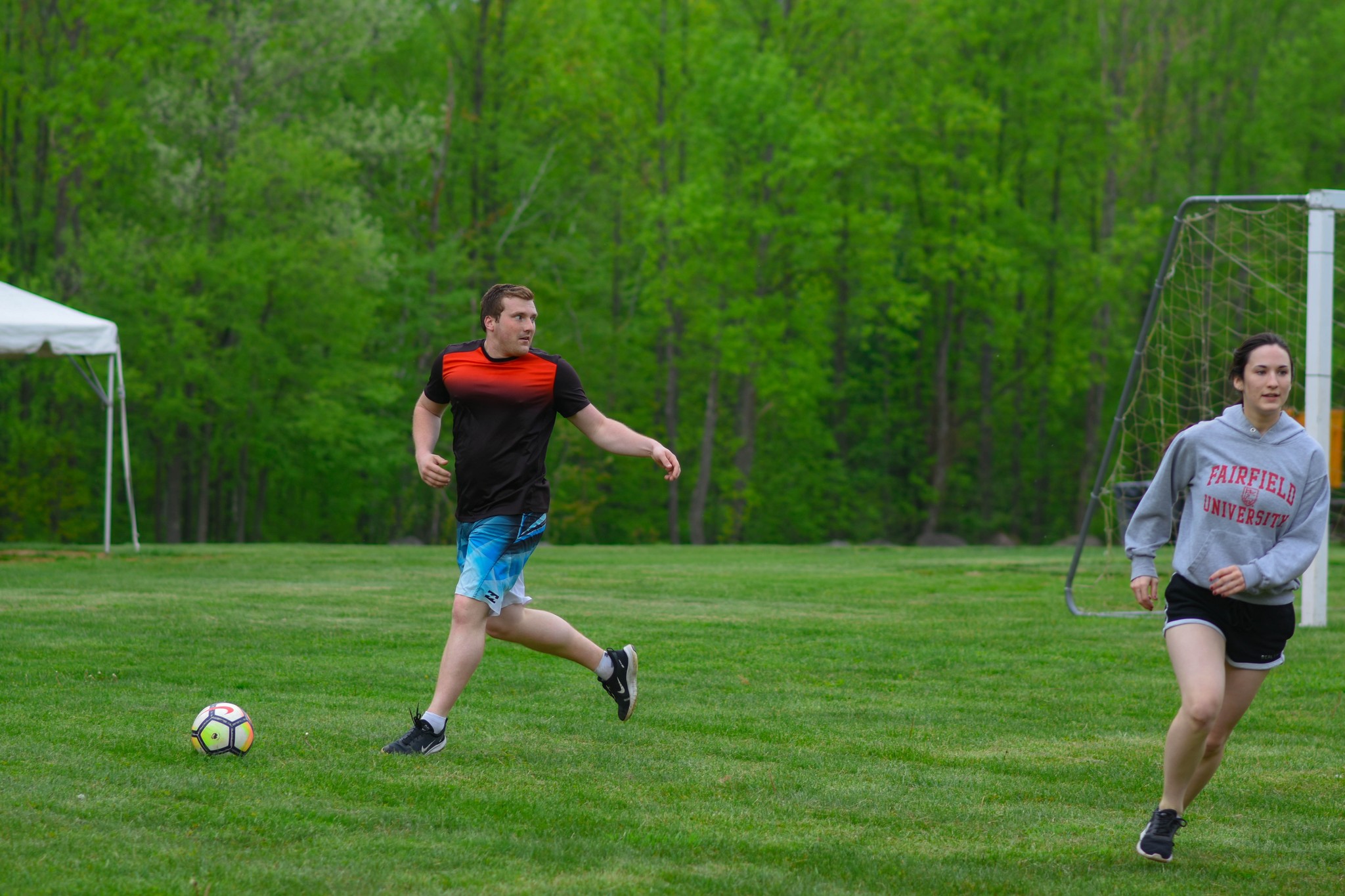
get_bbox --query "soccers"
[191,702,254,758]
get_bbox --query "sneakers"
[381,702,447,757]
[597,644,638,721]
[1136,808,1187,862]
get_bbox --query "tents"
[0,277,142,554]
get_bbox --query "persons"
[1123,333,1332,862]
[381,284,681,758]
